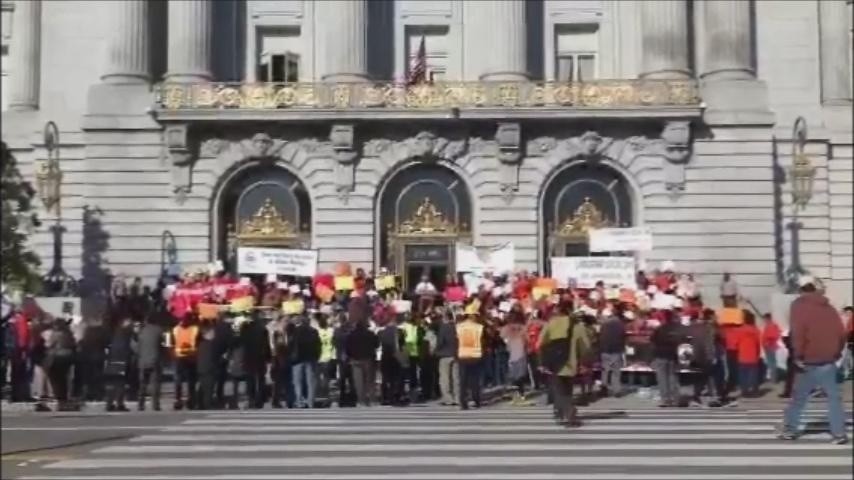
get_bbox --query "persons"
[0,268,854,426]
[774,276,848,444]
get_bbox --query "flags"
[408,38,426,85]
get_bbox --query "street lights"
[32,119,81,297]
[781,114,819,294]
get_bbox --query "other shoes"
[553,406,584,427]
[775,432,795,440]
[779,393,793,399]
[104,395,162,412]
[5,388,104,412]
[656,388,760,411]
[336,386,439,408]
[770,376,778,384]
[832,435,848,444]
[809,389,821,396]
[173,393,313,410]
[571,382,652,406]
[439,388,553,410]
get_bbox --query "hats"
[797,276,816,288]
